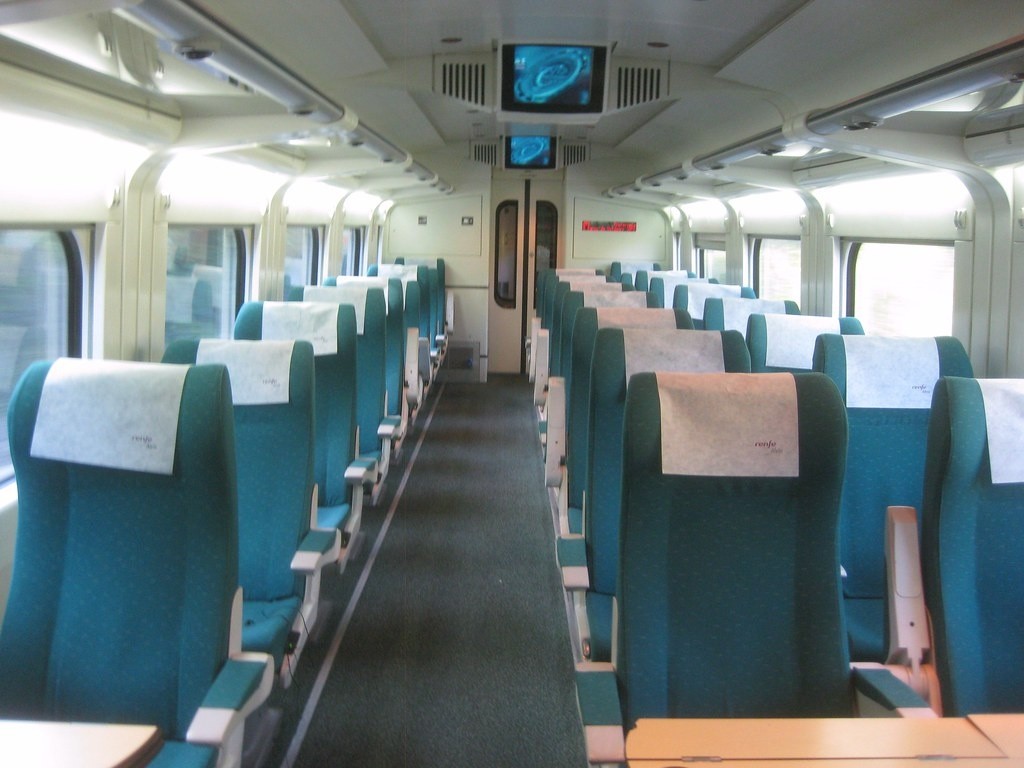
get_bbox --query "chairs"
[521,260,1024,768]
[0,256,454,767]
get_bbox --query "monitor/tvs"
[502,133,559,173]
[497,38,612,124]
